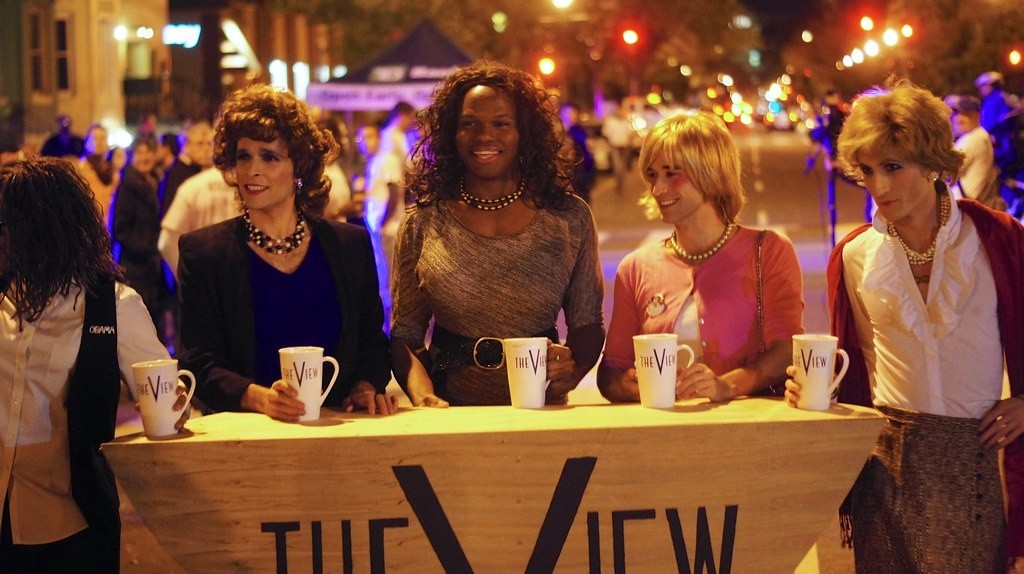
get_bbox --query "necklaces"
[670,222,735,260]
[460,172,526,210]
[887,194,951,264]
[244,208,306,254]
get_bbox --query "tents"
[343,17,473,85]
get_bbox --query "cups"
[632,333,695,406]
[131,359,196,438]
[279,345,340,423]
[791,333,849,409]
[503,337,560,409]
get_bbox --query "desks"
[99,392,886,574]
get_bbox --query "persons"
[122,114,160,151]
[39,114,85,159]
[784,73,1024,574]
[367,100,418,340]
[390,59,606,407]
[113,139,166,345]
[73,124,122,242]
[158,163,247,290]
[0,158,191,574]
[596,109,804,404]
[177,122,219,173]
[939,70,1024,215]
[161,132,196,215]
[176,84,398,423]
